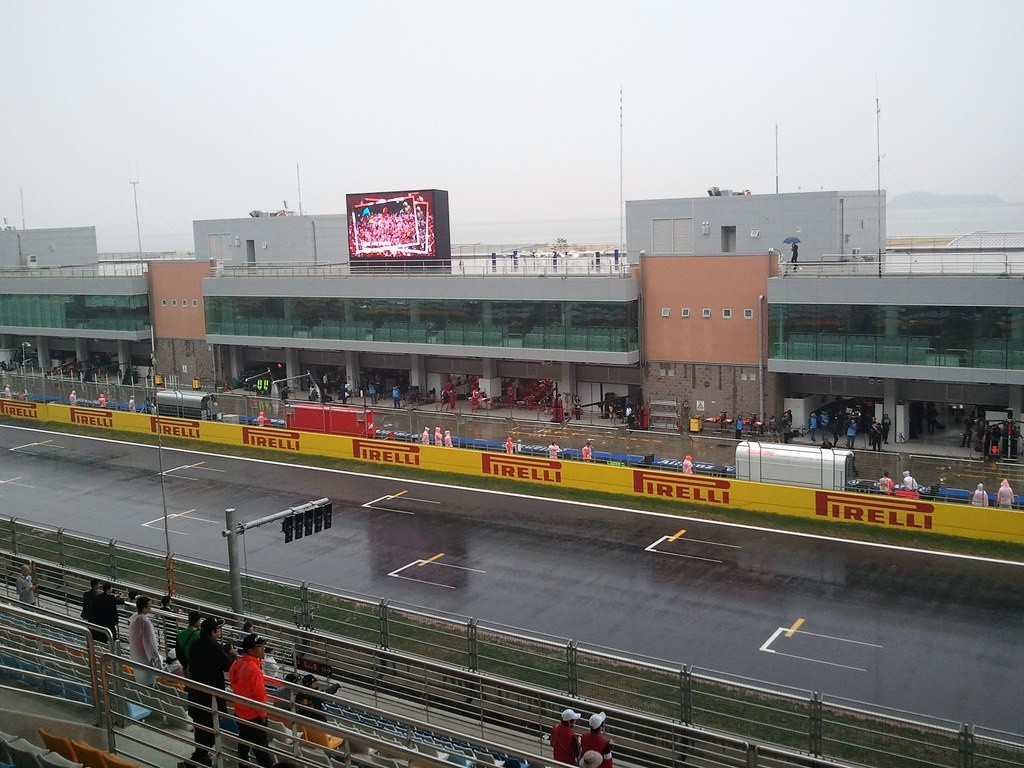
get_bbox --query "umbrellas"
[783,236,801,244]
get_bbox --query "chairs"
[0,588,534,768]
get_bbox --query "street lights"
[22,341,31,402]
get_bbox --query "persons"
[69,391,78,406]
[972,477,1014,510]
[581,441,593,463]
[16,564,39,610]
[718,401,1003,460]
[682,455,694,475]
[127,591,343,768]
[140,396,156,415]
[549,708,614,768]
[98,393,106,407]
[127,395,137,412]
[3,383,12,400]
[346,201,427,253]
[257,411,264,427]
[879,469,918,496]
[791,243,799,270]
[548,441,560,459]
[281,371,643,430]
[0,357,83,381]
[418,427,453,447]
[506,437,514,454]
[80,578,124,646]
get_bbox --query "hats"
[560,708,581,721]
[589,711,606,729]
[167,648,176,659]
[303,673,318,686]
[241,633,268,648]
[578,750,603,768]
[200,616,226,631]
[284,673,298,682]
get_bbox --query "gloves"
[333,683,341,688]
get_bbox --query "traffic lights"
[989,426,1001,456]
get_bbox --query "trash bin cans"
[192,378,200,389]
[690,415,704,431]
[156,373,162,384]
